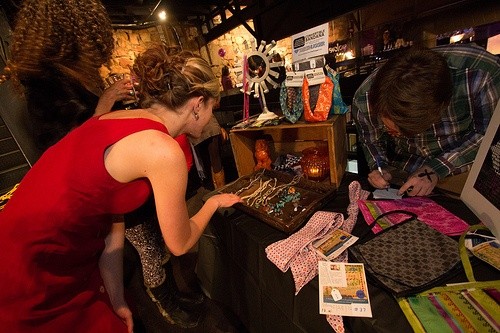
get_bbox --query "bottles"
[300,146,330,182]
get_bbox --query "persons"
[167,46,234,217]
[0,42,244,333]
[352,44,500,196]
[221,66,232,91]
[4,0,207,333]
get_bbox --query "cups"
[104,73,140,111]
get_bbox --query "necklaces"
[235,168,303,218]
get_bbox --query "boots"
[145,254,205,328]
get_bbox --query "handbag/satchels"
[348,209,477,298]
[357,195,471,237]
[397,225,500,333]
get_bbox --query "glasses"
[380,120,403,139]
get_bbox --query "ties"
[265,181,369,333]
[280,71,349,124]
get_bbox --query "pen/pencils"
[376,159,388,191]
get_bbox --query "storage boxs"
[230,112,348,189]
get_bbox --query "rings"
[376,173,380,176]
[130,92,134,95]
